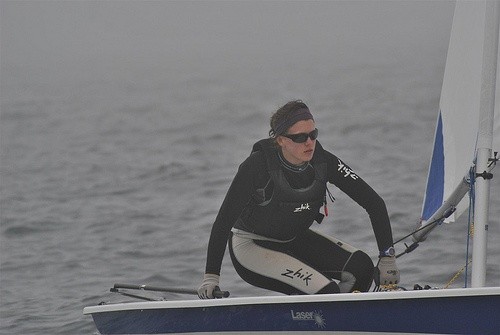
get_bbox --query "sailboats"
[83,0,499,335]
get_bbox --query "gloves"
[374,257,400,285]
[197,273,222,299]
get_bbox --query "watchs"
[379,247,396,258]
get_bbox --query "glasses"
[281,128,318,142]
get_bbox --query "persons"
[196,101,400,300]
